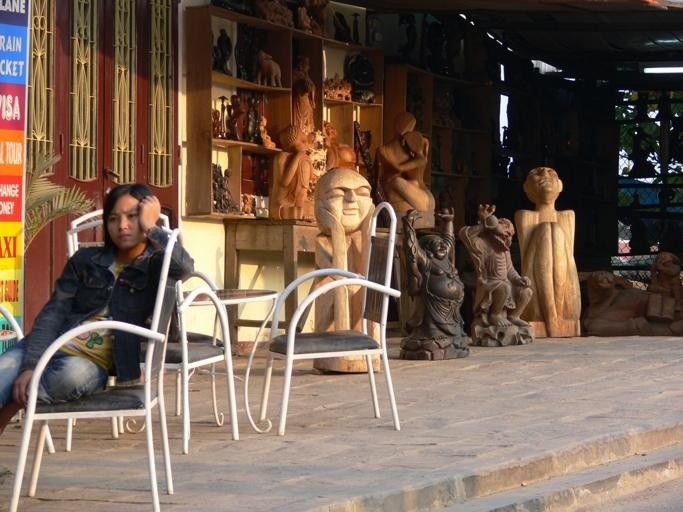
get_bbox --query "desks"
[183,289,279,433]
[224,216,392,354]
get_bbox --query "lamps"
[184,6,385,222]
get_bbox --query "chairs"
[11,226,180,512]
[0,300,57,454]
[260,202,403,435]
[64,210,238,454]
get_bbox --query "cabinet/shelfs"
[384,65,496,241]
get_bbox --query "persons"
[1,184,195,415]
[376,111,436,229]
[514,166,583,337]
[646,251,683,321]
[402,207,469,340]
[312,168,381,373]
[213,169,233,209]
[268,125,316,222]
[458,205,534,326]
[292,55,317,127]
